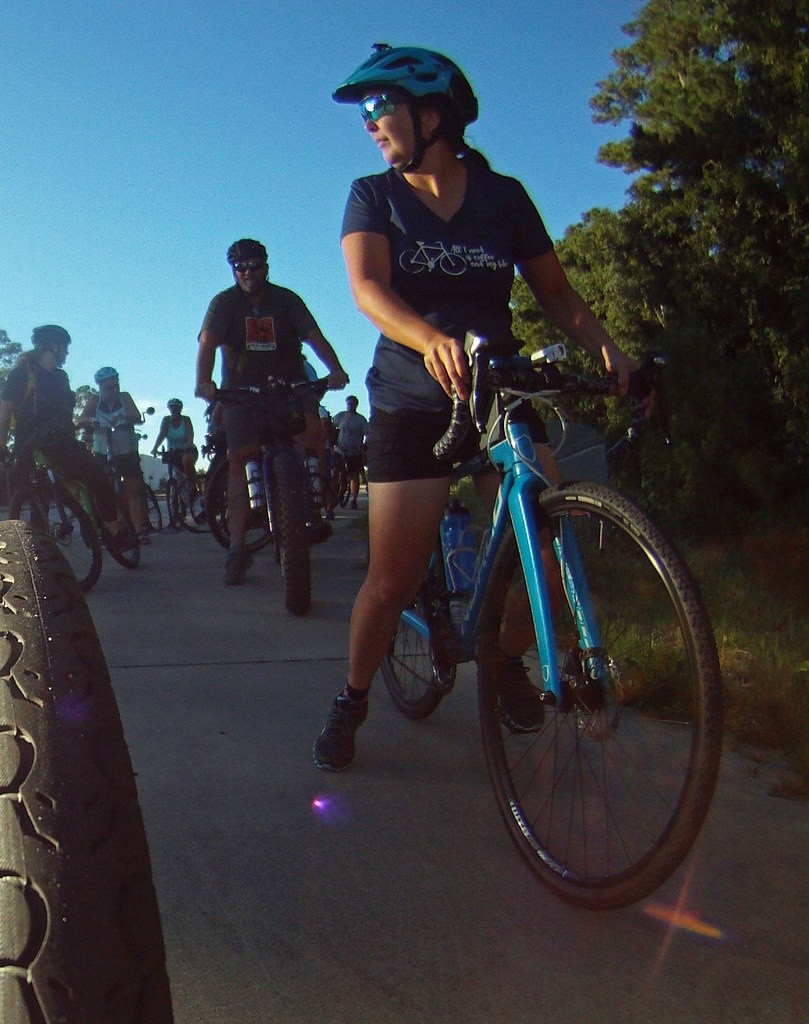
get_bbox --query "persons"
[0,325,134,553]
[151,399,197,528]
[314,48,656,772]
[333,396,369,510]
[79,367,158,546]
[194,239,348,587]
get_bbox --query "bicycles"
[364,344,723,910]
[195,376,332,616]
[0,393,354,1024]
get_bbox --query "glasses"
[358,91,410,122]
[233,259,266,272]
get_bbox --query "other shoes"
[140,536,150,543]
[188,484,199,503]
[349,502,358,510]
[114,528,130,549]
[325,511,335,520]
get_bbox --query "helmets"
[345,395,359,406]
[95,367,119,386]
[226,239,268,265]
[167,398,183,410]
[332,43,479,122]
[31,325,71,346]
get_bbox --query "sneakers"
[312,694,369,771]
[308,521,332,548]
[224,546,253,584]
[495,657,546,732]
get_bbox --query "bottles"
[302,451,323,505]
[245,457,265,511]
[441,500,476,590]
